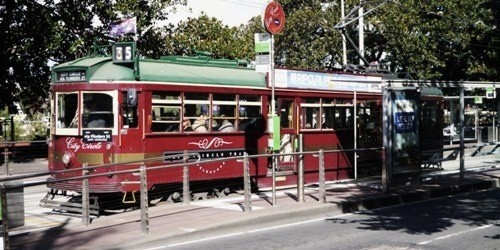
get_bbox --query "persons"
[172,106,375,132]
[84,97,114,129]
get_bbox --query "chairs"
[60,96,441,141]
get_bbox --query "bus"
[37,37,389,216]
